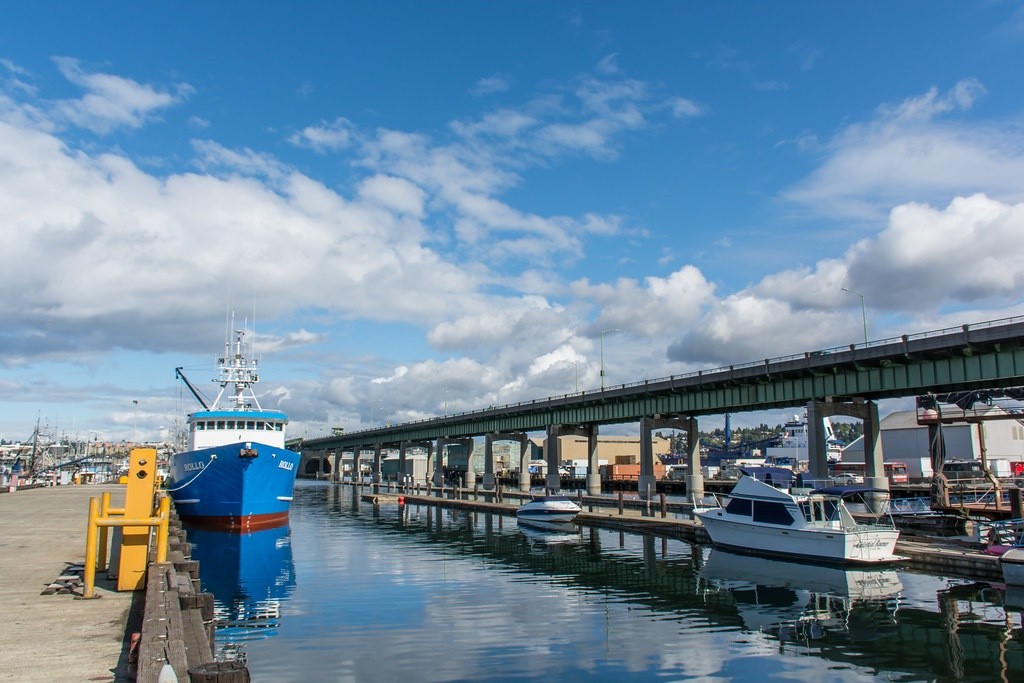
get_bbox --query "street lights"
[843,287,868,352]
[443,376,459,418]
[565,360,578,393]
[600,328,621,391]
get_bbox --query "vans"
[942,458,989,486]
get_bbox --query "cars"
[831,472,864,485]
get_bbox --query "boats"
[737,464,1024,587]
[516,516,581,545]
[515,484,582,522]
[173,518,299,665]
[0,405,130,495]
[163,287,302,534]
[690,538,905,657]
[690,471,910,567]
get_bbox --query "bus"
[834,461,909,486]
